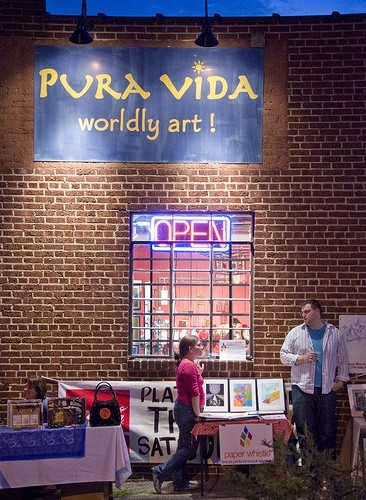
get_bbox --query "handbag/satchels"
[89,382,121,426]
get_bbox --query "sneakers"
[174,483,200,491]
[152,467,162,494]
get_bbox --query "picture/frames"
[229,378,257,413]
[133,285,140,310]
[132,316,140,341]
[132,345,139,355]
[256,377,286,412]
[202,379,229,413]
[347,384,366,417]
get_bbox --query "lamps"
[195,0,219,47]
[69,0,93,45]
[160,278,168,305]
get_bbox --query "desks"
[0,422,133,500]
[340,417,366,485]
[191,420,294,496]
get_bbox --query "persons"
[151,335,212,493]
[10,375,57,500]
[280,299,350,465]
